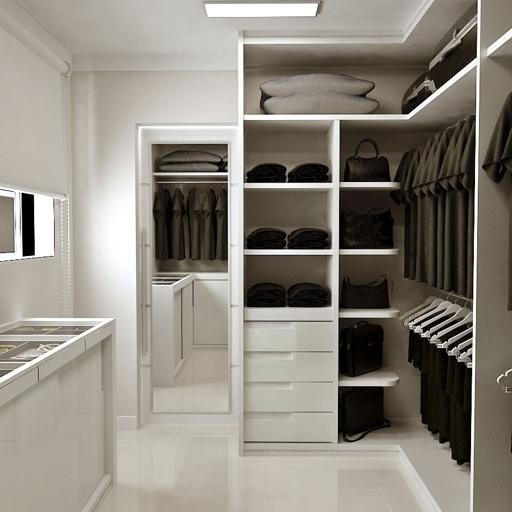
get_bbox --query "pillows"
[156,149,228,171]
[261,95,381,113]
[258,71,377,97]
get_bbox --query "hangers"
[495,368,511,395]
[395,287,475,373]
[161,182,226,190]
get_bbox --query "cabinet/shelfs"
[404,0,511,512]
[0,315,120,511]
[152,270,195,388]
[236,31,405,455]
[148,139,230,351]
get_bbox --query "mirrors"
[0,186,56,264]
[133,120,237,431]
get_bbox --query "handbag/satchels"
[339,386,392,444]
[343,137,392,182]
[339,271,390,310]
[340,320,384,379]
[340,205,394,250]
